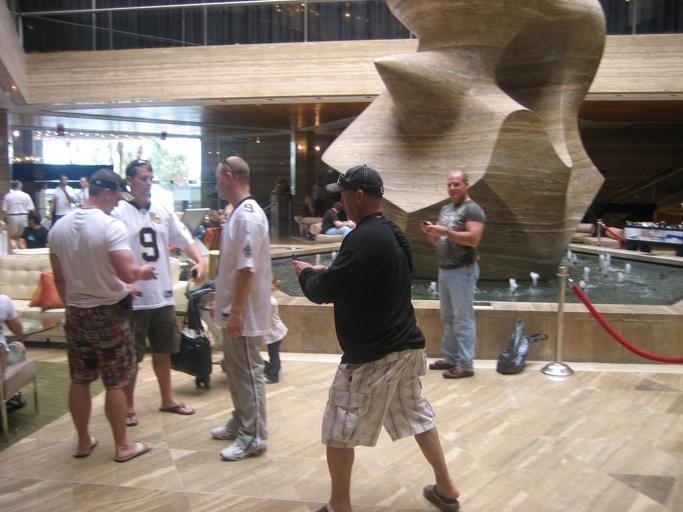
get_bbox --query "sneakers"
[220,443,267,462]
[7,392,26,407]
[211,425,237,440]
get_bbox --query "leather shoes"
[430,359,457,370]
[443,368,473,378]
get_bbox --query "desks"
[1,315,59,347]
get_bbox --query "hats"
[325,164,384,195]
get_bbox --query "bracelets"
[440,229,449,238]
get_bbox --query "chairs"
[1,341,40,436]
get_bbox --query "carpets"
[0,359,106,453]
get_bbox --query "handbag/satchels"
[171,300,211,376]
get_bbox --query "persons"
[110,160,205,425]
[321,201,355,239]
[207,156,289,462]
[2,294,28,413]
[298,195,323,239]
[421,167,486,379]
[50,169,159,463]
[293,164,460,511]
[1,175,90,249]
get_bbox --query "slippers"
[72,436,96,457]
[424,484,459,512]
[113,441,151,462]
[127,411,138,426]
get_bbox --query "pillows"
[39,272,67,310]
[27,277,46,306]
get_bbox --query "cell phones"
[420,221,430,225]
[192,270,197,278]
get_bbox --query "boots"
[160,401,195,415]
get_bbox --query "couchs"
[309,220,345,245]
[1,251,192,343]
